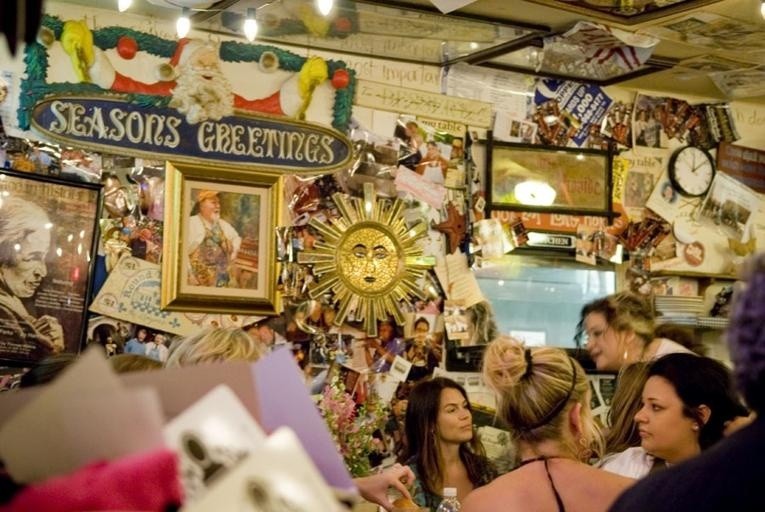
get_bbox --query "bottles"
[435,487,461,512]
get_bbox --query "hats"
[198,189,219,202]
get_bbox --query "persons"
[406,315,441,378]
[390,376,497,512]
[395,119,449,181]
[611,252,765,512]
[0,196,66,367]
[60,20,330,126]
[106,327,168,363]
[186,189,241,289]
[601,353,747,486]
[354,461,416,512]
[581,360,677,482]
[460,334,639,512]
[574,289,701,386]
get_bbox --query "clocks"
[669,145,715,197]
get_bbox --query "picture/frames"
[161,159,284,315]
[0,168,105,369]
[479,131,621,225]
